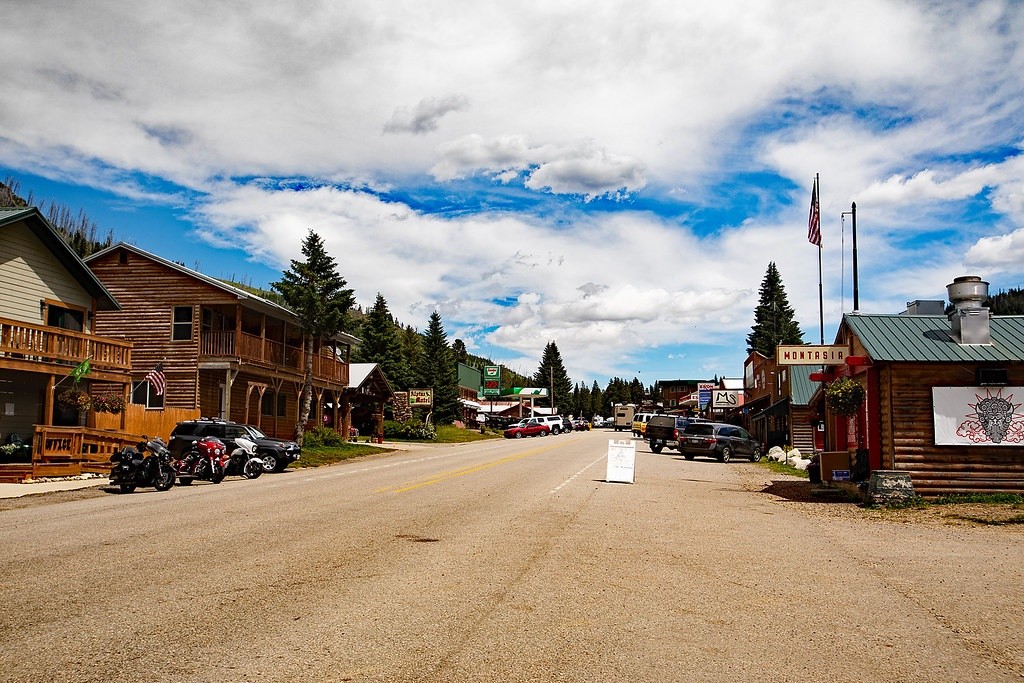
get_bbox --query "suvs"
[167,418,302,474]
[677,422,763,464]
[508,415,563,435]
[644,414,713,455]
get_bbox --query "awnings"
[750,396,788,422]
[726,394,769,417]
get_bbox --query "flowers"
[58,382,91,417]
[93,384,126,415]
[823,377,866,417]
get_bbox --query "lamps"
[88,311,94,320]
[40,300,47,310]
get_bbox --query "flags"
[807,179,823,248]
[144,360,165,396]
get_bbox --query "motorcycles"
[110,434,179,493]
[172,435,231,486]
[110,446,140,475]
[222,435,263,479]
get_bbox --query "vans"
[632,413,659,438]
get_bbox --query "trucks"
[614,405,636,432]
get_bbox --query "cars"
[503,422,550,439]
[561,416,614,434]
